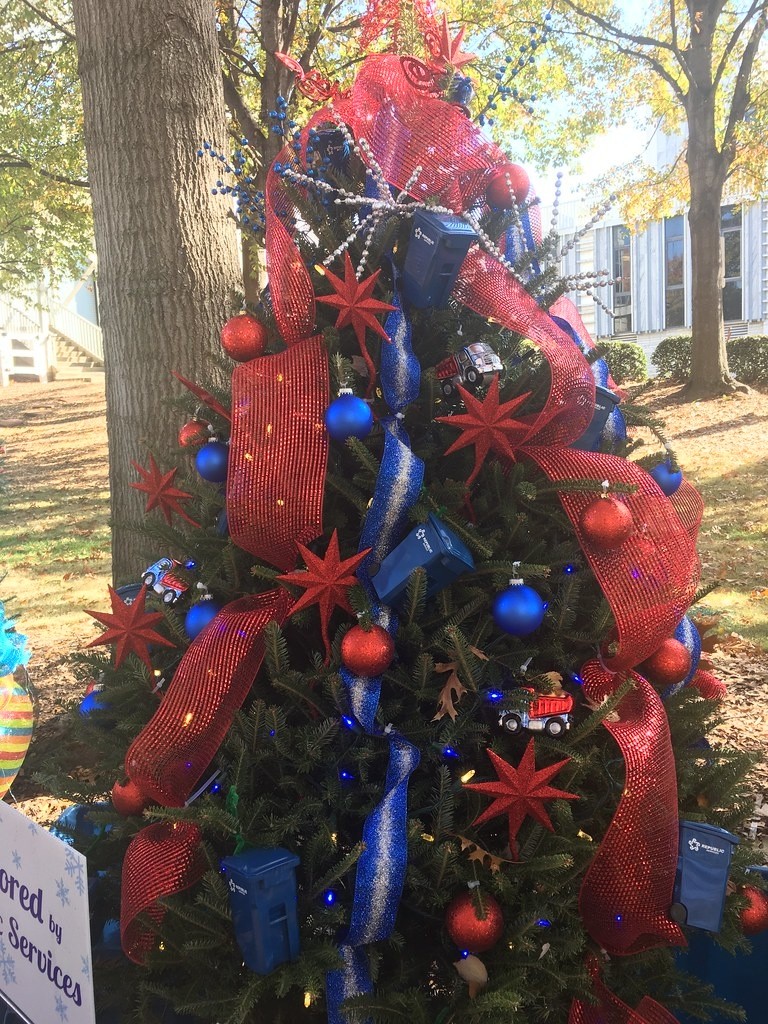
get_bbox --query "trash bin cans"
[563,382,621,451]
[219,846,302,978]
[308,127,351,208]
[398,209,481,312]
[671,817,742,936]
[115,583,156,656]
[367,512,479,618]
